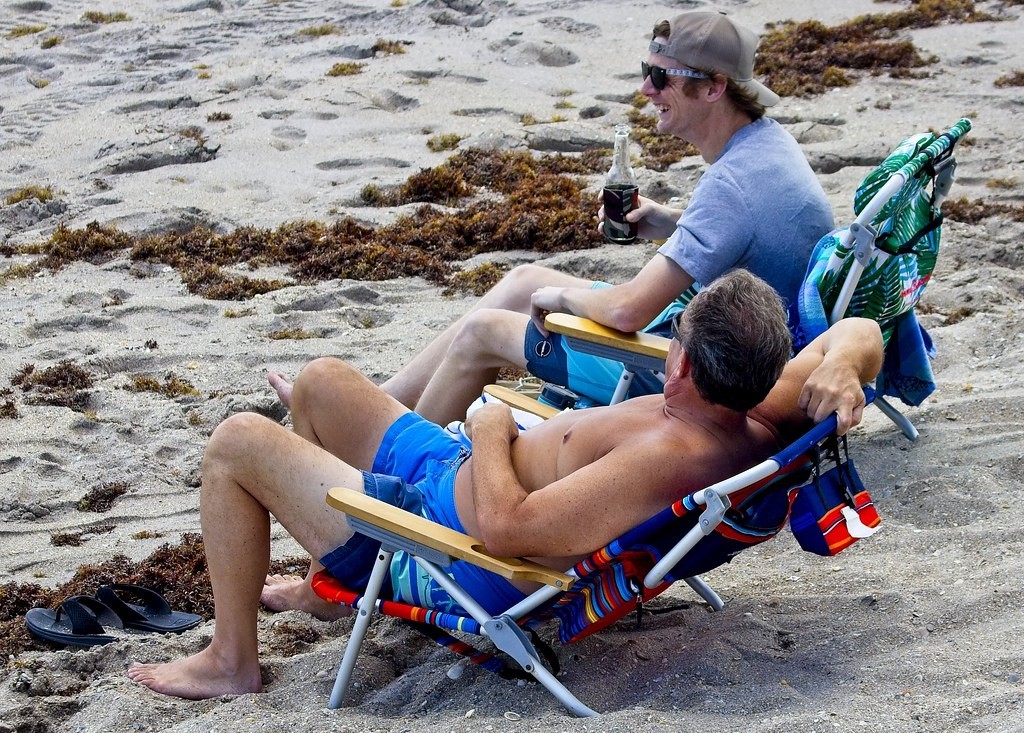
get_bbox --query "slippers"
[24,594,149,648]
[90,583,204,634]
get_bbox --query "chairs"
[311,382,877,719]
[541,118,972,441]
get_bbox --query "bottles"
[603,124,639,244]
[538,382,578,412]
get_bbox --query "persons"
[268,9,836,429]
[127,268,883,700]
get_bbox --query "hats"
[650,10,781,107]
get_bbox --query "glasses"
[642,60,712,90]
